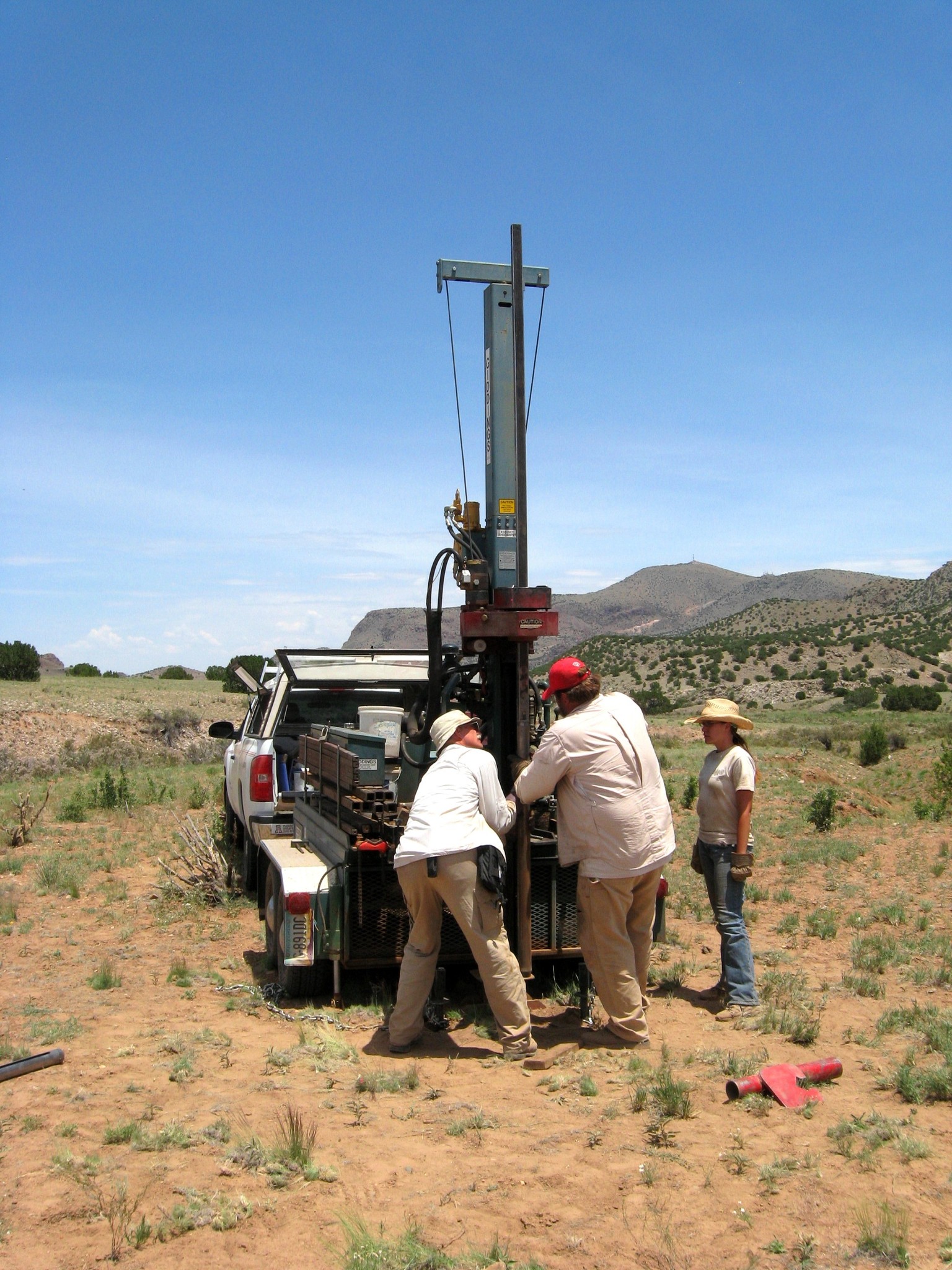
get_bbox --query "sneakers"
[715,1002,761,1022]
[699,985,727,1001]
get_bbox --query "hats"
[428,710,483,757]
[683,697,755,730]
[541,656,591,701]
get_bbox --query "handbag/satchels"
[477,845,507,895]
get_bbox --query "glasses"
[457,721,480,733]
[698,719,724,727]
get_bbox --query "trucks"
[206,213,667,1018]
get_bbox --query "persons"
[512,655,677,1048]
[386,707,541,1059]
[691,698,761,1020]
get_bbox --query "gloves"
[690,843,703,875]
[507,753,532,783]
[730,852,755,883]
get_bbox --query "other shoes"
[503,1038,538,1062]
[389,1031,426,1053]
[581,1024,651,1050]
